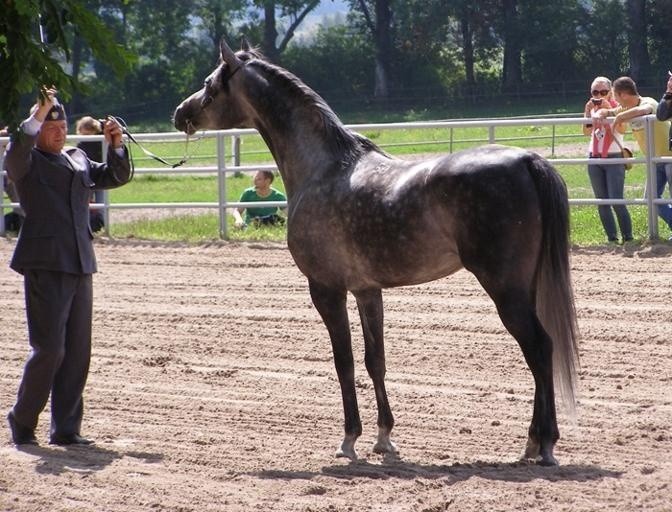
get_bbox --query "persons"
[0,116,104,232]
[233,171,288,230]
[4,84,130,445]
[583,75,672,242]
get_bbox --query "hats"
[30,99,69,121]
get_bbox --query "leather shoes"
[6,411,41,447]
[50,431,95,447]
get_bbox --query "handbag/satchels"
[620,147,634,169]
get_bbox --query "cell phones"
[592,99,602,106]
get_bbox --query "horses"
[172,32,581,470]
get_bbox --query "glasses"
[591,89,611,96]
